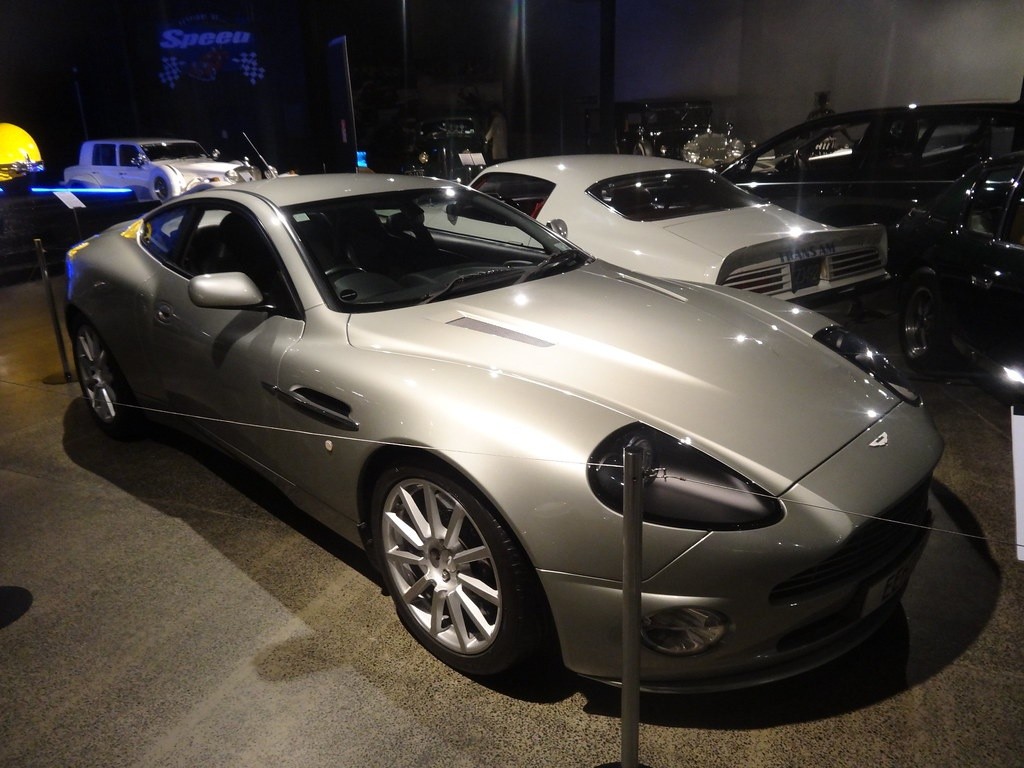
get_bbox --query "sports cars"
[57,172,945,696]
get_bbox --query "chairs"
[611,187,655,215]
[199,213,282,295]
[328,208,430,276]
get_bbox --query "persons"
[807,91,856,144]
[485,107,508,162]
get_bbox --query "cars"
[1,171,162,275]
[62,138,235,202]
[884,150,1023,399]
[376,154,890,305]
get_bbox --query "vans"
[713,102,1023,233]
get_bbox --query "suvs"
[585,102,710,162]
[414,118,487,169]
